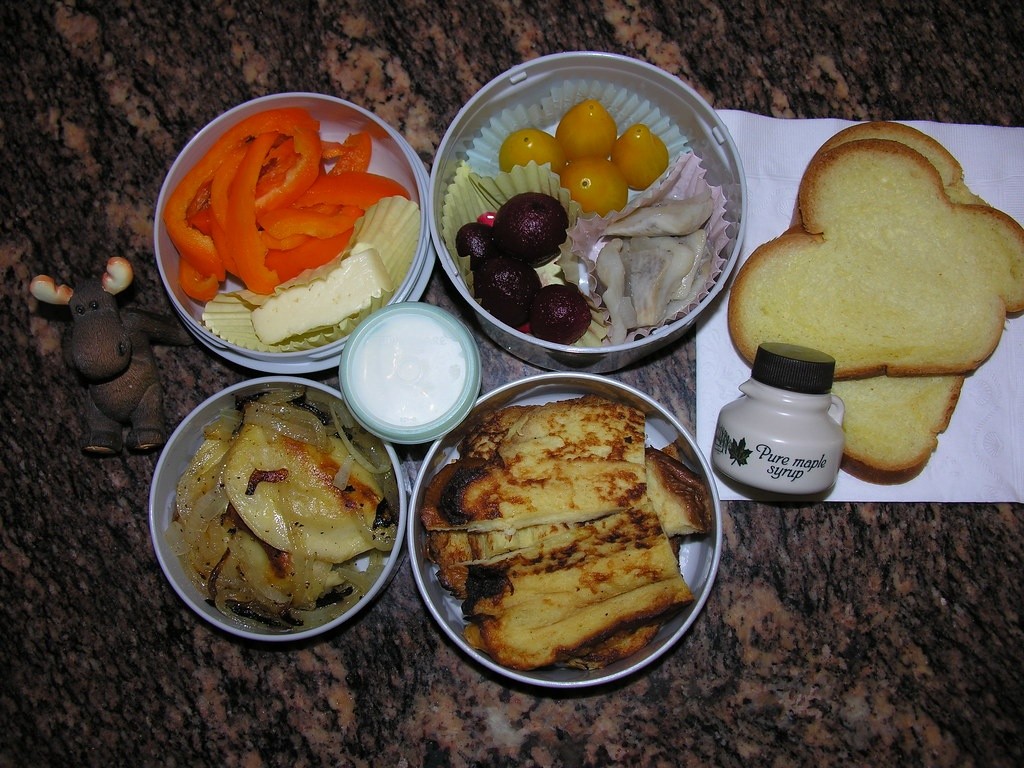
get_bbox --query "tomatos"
[499,97,670,216]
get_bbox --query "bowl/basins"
[152,91,431,363]
[431,51,746,374]
[409,373,721,687]
[148,377,410,642]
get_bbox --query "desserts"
[250,246,394,344]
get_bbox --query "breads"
[727,121,1024,476]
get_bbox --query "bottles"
[713,342,845,494]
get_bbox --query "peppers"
[163,106,414,302]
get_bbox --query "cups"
[339,303,483,446]
